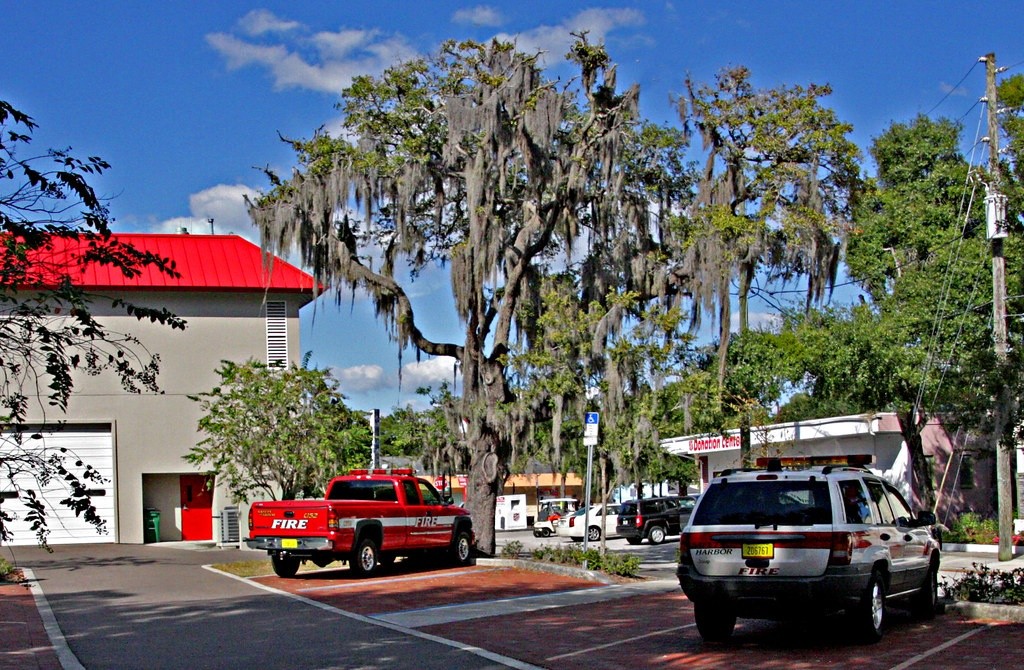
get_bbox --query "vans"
[616,496,697,546]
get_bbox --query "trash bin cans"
[144,507,161,544]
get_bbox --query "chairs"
[537,506,870,525]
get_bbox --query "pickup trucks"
[674,463,945,647]
[240,468,478,579]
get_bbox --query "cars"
[556,503,624,542]
